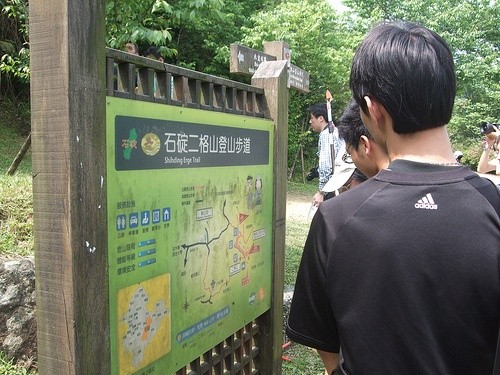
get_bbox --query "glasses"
[342,133,365,164]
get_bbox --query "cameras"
[480,121,500,135]
[306,166,319,181]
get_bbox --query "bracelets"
[484,147,490,151]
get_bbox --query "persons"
[309,102,500,208]
[286,22,500,375]
